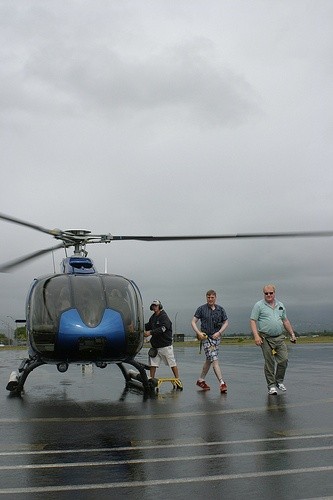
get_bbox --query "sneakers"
[268,387,277,395]
[277,383,286,391]
[220,384,227,393]
[197,380,210,390]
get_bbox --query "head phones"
[150,303,162,310]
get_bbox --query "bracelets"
[291,334,295,336]
[218,331,221,337]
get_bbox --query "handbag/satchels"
[148,348,158,358]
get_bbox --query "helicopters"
[1,214,333,402]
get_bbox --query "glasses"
[263,291,273,295]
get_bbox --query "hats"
[151,300,160,305]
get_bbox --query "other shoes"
[174,383,183,391]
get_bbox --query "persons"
[192,290,228,393]
[143,299,183,390]
[250,285,297,395]
